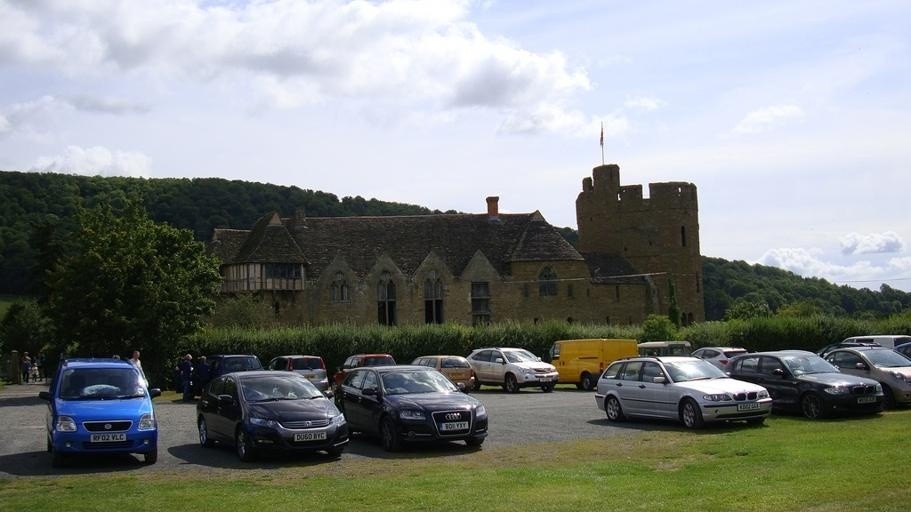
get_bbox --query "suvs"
[468,346,559,393]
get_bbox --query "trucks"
[548,337,639,388]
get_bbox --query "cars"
[37,352,159,464]
[411,354,475,393]
[197,370,348,465]
[270,354,330,394]
[333,354,396,392]
[337,365,489,453]
[192,354,264,399]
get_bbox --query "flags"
[600,127,604,145]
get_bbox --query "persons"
[21,352,42,384]
[197,356,212,396]
[128,351,145,379]
[176,354,194,401]
